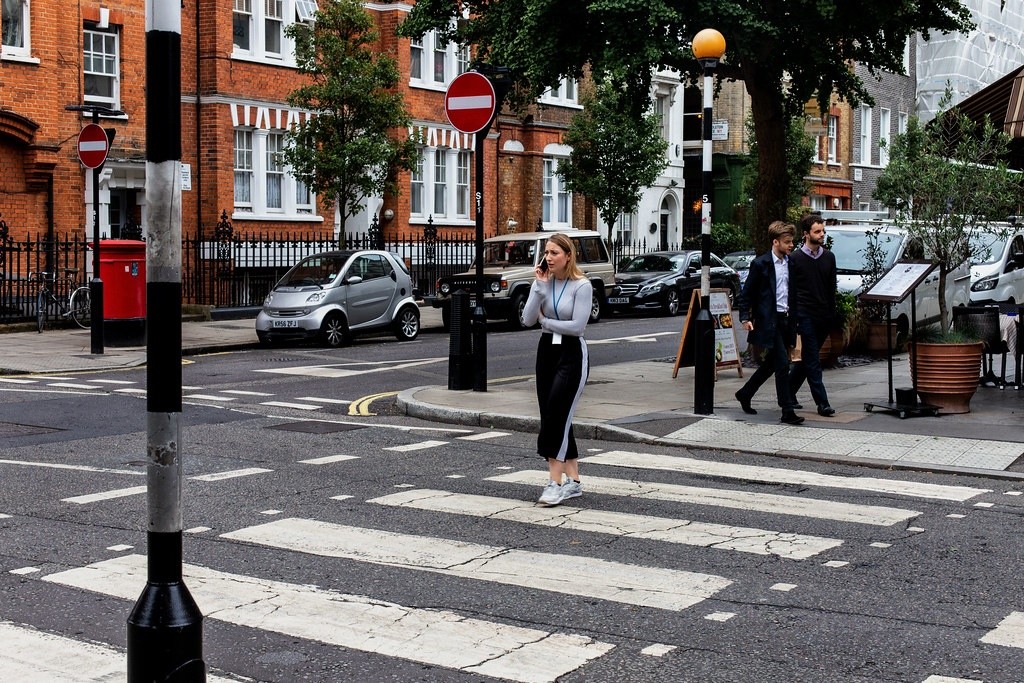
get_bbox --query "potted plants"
[872,78,1024,414]
[801,225,898,365]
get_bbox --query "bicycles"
[28,267,91,334]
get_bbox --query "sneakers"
[538,482,565,506]
[560,477,583,499]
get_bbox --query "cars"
[721,208,1024,350]
[601,249,741,319]
[253,248,422,349]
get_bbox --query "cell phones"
[539,254,548,273]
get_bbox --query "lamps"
[834,198,840,210]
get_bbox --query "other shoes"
[735,389,758,414]
[780,412,805,425]
[817,403,835,415]
[791,393,803,409]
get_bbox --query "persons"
[735,220,805,424]
[523,234,592,505]
[789,216,838,417]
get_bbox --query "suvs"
[431,227,619,333]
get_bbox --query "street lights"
[688,28,725,417]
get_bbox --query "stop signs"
[76,123,109,169]
[443,72,496,135]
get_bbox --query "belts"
[777,312,790,317]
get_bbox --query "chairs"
[951,306,1010,389]
